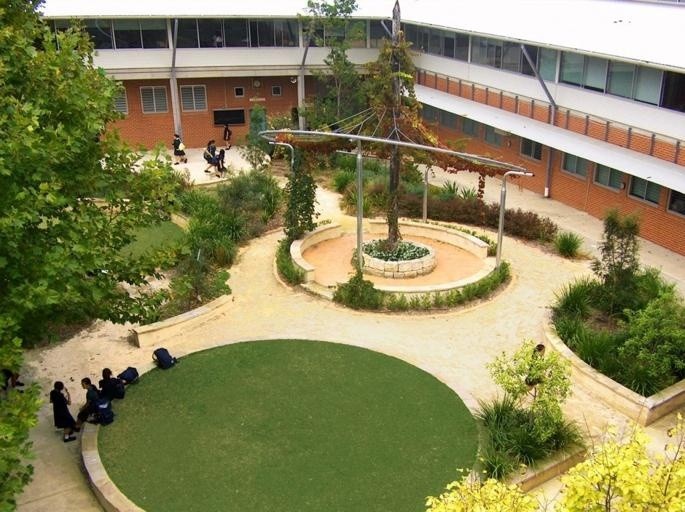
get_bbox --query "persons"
[172,134,187,165]
[99,368,127,395]
[78,377,100,423]
[49,381,81,443]
[223,124,232,150]
[204,140,226,179]
[525,344,545,389]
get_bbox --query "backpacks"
[152,348,177,369]
[85,367,139,424]
[178,142,185,151]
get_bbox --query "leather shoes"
[64,426,81,442]
